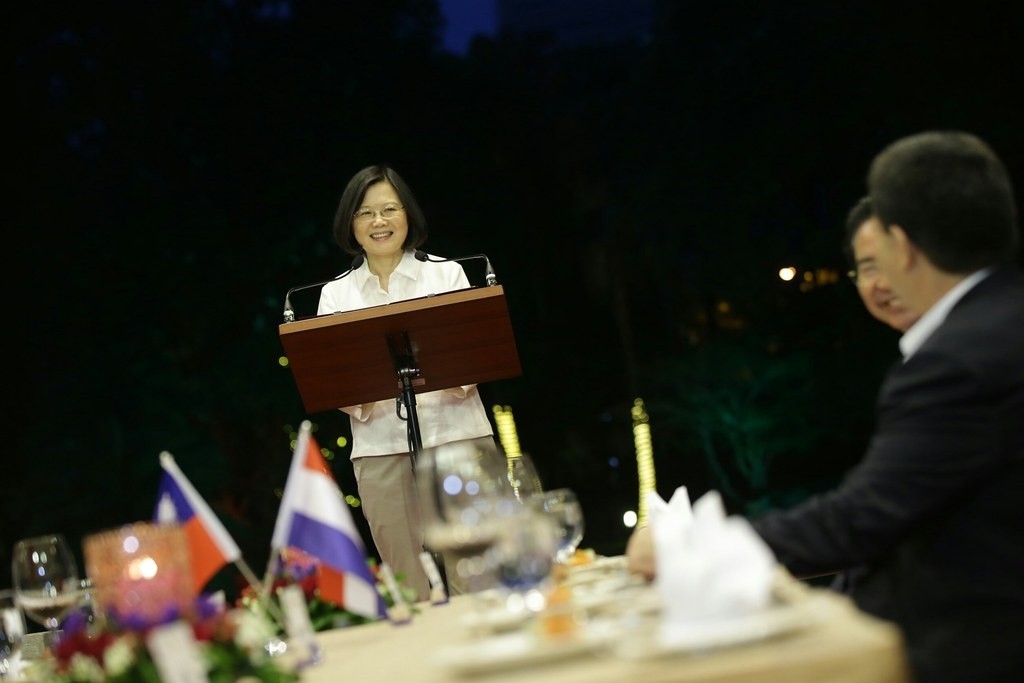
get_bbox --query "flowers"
[52,624,331,683]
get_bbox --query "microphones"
[414,250,497,287]
[282,256,364,322]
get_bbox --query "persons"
[750,131,1024,683]
[316,165,528,603]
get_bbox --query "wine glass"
[402,441,528,630]
[521,489,587,636]
[12,534,78,656]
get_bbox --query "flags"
[153,458,242,597]
[271,431,387,620]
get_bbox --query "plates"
[437,617,620,674]
[620,598,838,659]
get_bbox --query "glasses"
[848,265,881,287]
[352,205,404,222]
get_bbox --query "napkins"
[652,488,781,629]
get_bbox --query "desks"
[240,554,902,683]
